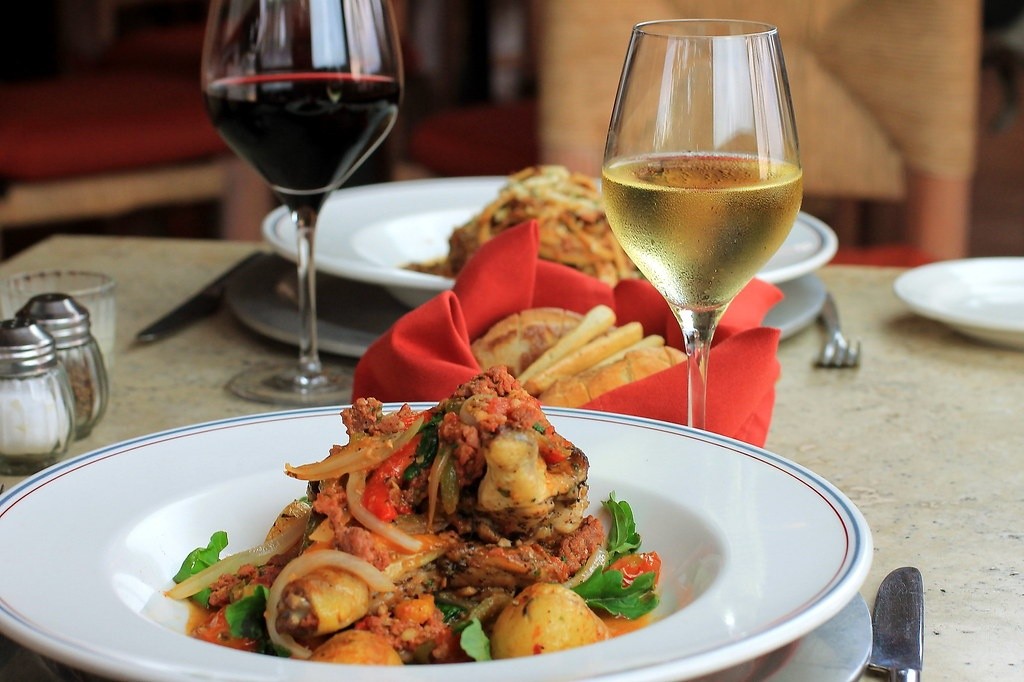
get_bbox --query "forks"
[816,291,863,367]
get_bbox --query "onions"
[163,415,455,657]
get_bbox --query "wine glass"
[601,19,804,431]
[196,1,404,403]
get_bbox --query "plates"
[1,591,872,682]
[224,253,828,358]
[893,255,1024,350]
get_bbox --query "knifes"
[868,566,925,681]
[134,249,265,346]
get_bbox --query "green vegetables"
[173,404,659,665]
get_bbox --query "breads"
[468,305,585,376]
[535,343,688,411]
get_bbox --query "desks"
[0,231,1024,682]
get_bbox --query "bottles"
[0,316,76,476]
[14,292,109,440]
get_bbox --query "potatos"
[304,630,403,666]
[492,582,610,659]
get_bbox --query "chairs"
[534,0,988,272]
[0,61,275,237]
[62,0,212,70]
[389,0,540,179]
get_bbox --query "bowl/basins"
[259,174,838,311]
[0,404,874,681]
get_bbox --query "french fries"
[516,305,665,394]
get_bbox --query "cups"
[0,269,118,376]
